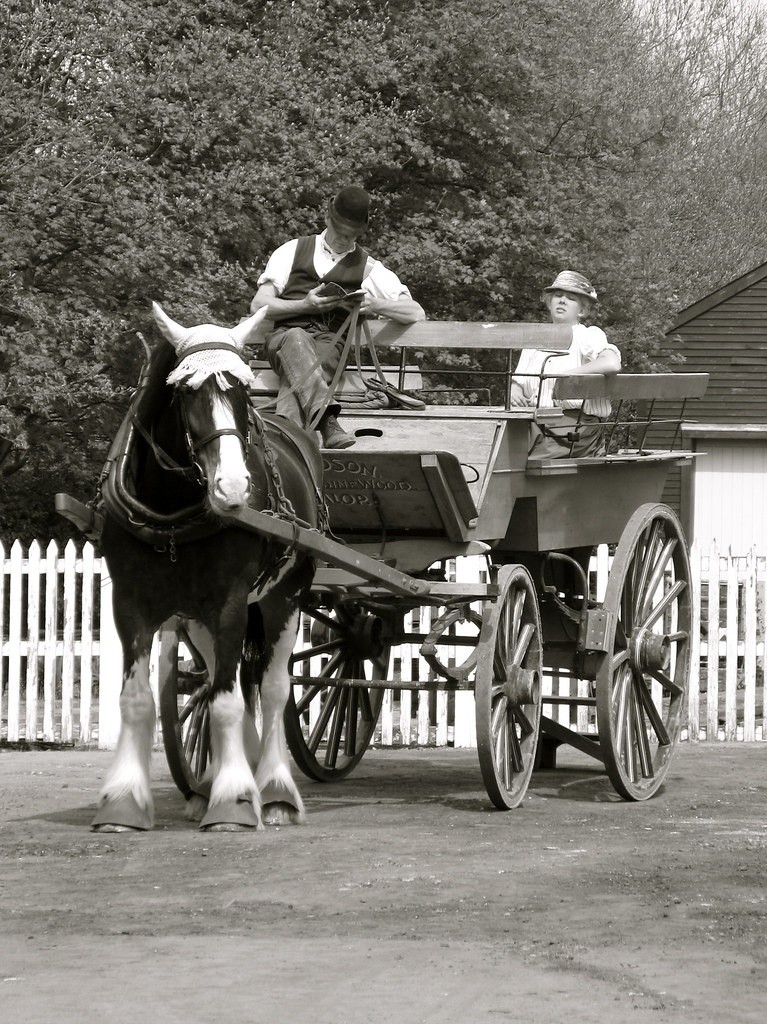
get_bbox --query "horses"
[92,300,325,835]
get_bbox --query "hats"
[328,186,369,236]
[544,270,599,303]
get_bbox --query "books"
[319,282,368,302]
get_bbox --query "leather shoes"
[321,414,356,449]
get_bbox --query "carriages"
[52,299,715,835]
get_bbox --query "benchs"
[238,316,573,421]
[422,371,710,457]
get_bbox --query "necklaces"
[248,186,427,450]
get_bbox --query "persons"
[506,269,623,459]
